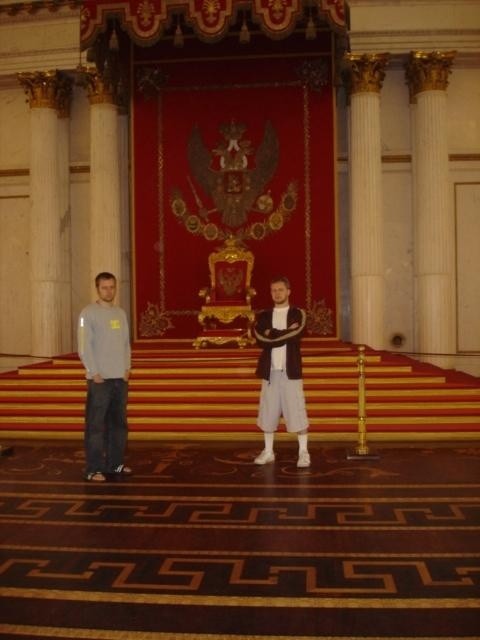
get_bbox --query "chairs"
[190,235,262,351]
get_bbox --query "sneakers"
[254,450,275,465]
[297,453,311,468]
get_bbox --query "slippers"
[80,471,107,482]
[104,464,131,475]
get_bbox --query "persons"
[251,275,312,468]
[76,272,134,482]
